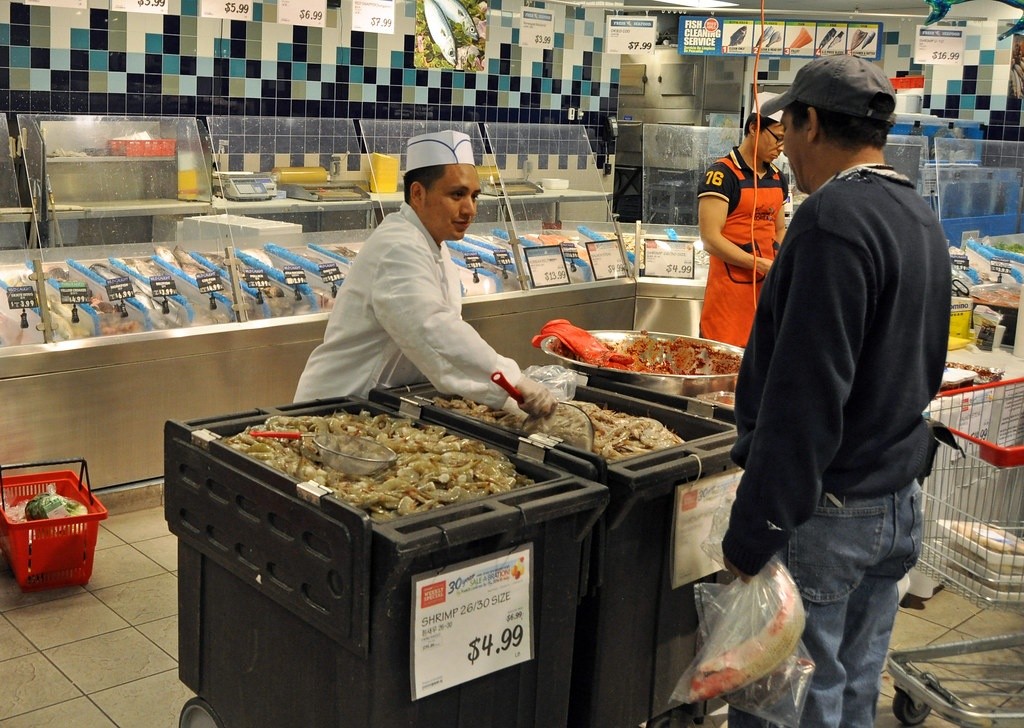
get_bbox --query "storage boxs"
[163,383,740,728]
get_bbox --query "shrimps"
[231,410,534,518]
[429,379,687,463]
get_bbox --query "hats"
[759,53,898,128]
[751,91,783,123]
[403,130,477,176]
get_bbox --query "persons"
[721,55,951,728]
[293,130,557,420]
[699,93,788,346]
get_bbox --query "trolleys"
[883,375,1024,728]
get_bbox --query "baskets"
[0,456,109,593]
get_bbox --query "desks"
[908,347,1024,599]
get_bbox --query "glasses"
[765,126,784,147]
